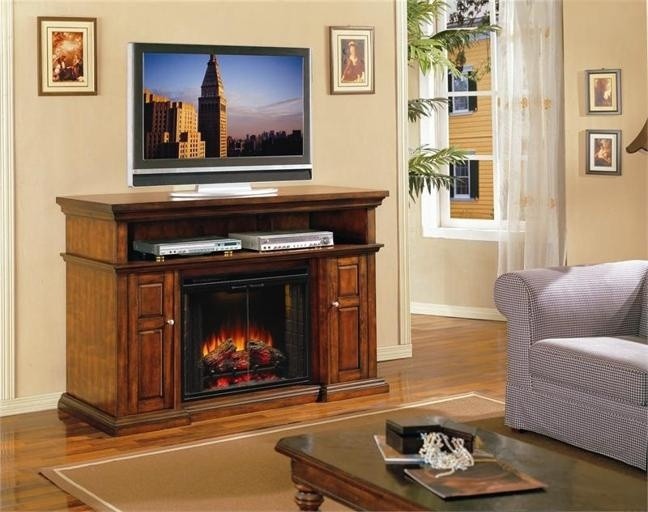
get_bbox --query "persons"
[595,139,611,164]
[342,41,364,81]
[595,79,611,107]
[72,54,83,80]
[53,55,66,81]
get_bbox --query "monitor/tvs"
[126,41,313,198]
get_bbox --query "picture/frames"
[325,23,376,96]
[35,14,99,97]
[585,69,623,176]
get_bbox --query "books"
[373,432,497,463]
[402,457,550,500]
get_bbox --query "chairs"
[498,257,648,472]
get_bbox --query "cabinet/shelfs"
[59,182,393,439]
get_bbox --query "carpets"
[38,389,648,512]
